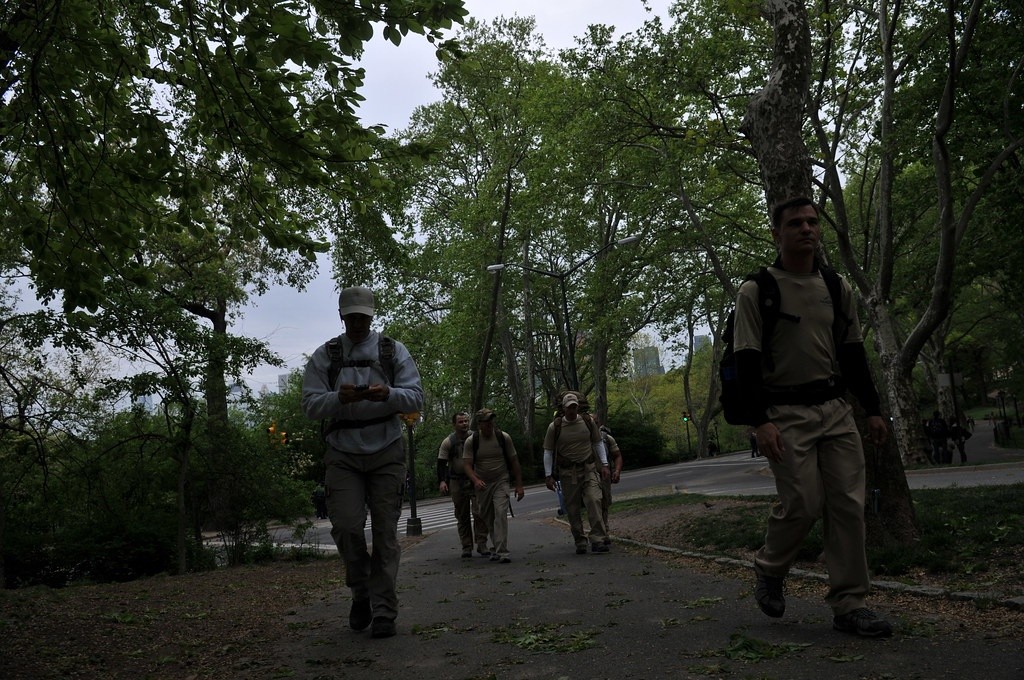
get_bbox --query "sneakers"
[833,607,891,638]
[754,568,787,619]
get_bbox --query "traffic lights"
[683,412,687,422]
[267,423,276,434]
[280,432,288,444]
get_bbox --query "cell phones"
[354,384,369,392]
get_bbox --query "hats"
[562,393,579,407]
[476,408,497,423]
[339,286,375,318]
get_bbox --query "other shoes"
[490,554,511,563]
[370,616,396,638]
[350,587,372,630]
[592,542,610,552]
[462,549,472,557]
[477,543,491,555]
[576,546,587,554]
[605,538,611,545]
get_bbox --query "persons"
[749,431,761,459]
[930,410,949,461]
[544,394,622,553]
[730,199,893,638]
[437,412,491,557]
[948,416,967,463]
[461,407,525,563]
[303,285,423,636]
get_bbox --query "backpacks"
[719,267,845,426]
[599,425,613,462]
[552,391,596,482]
[929,419,944,437]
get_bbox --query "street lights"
[488,235,637,394]
